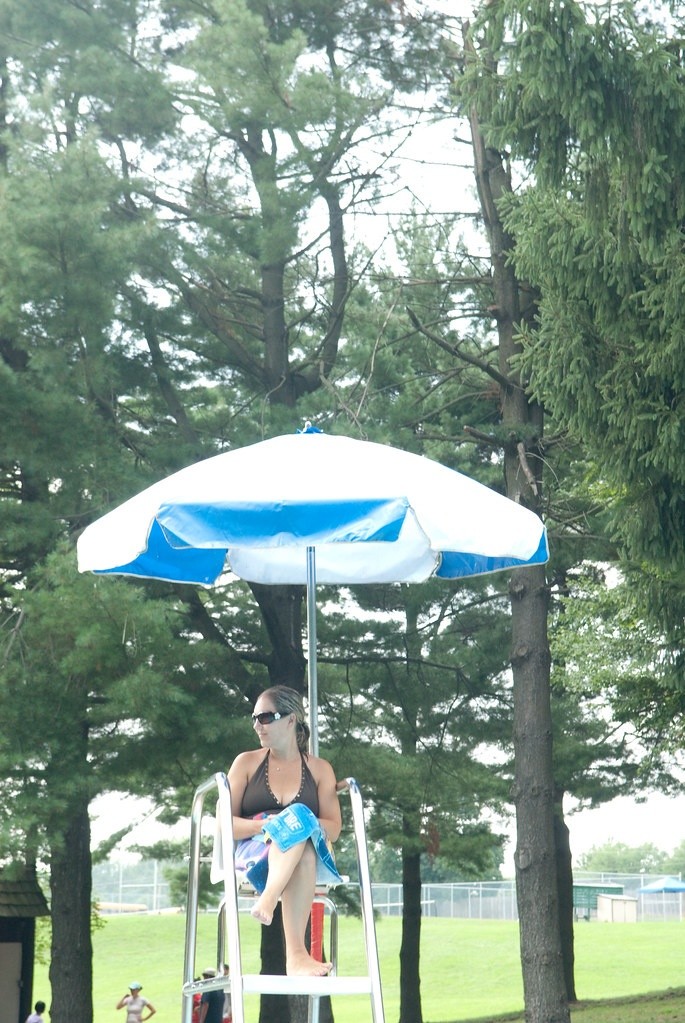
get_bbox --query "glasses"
[251,711,290,725]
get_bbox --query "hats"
[129,983,142,989]
[202,968,216,977]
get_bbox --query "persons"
[223,685,343,975]
[26,1001,46,1022]
[222,964,233,1023]
[200,968,223,1023]
[192,977,202,1023]
[116,982,156,1023]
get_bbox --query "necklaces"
[271,754,286,771]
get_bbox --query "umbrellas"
[635,876,685,918]
[77,419,551,759]
